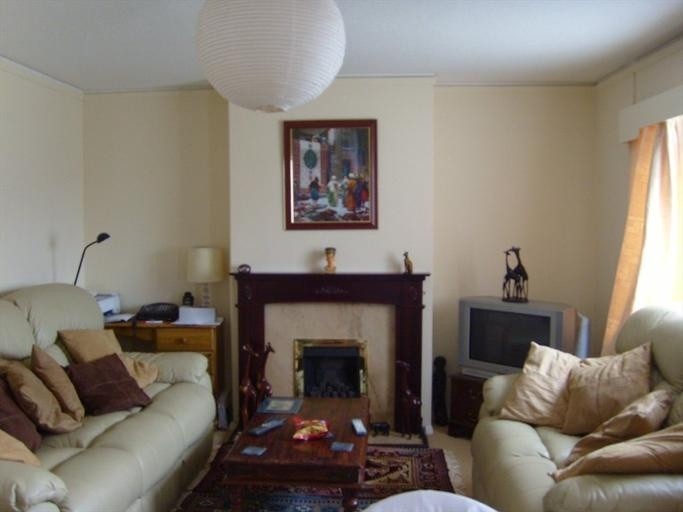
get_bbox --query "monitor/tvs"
[458,297,590,379]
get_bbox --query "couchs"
[1,284,216,512]
[470,306,682,512]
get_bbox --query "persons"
[307,171,369,213]
[322,245,335,272]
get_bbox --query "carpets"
[176,429,456,512]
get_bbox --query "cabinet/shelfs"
[447,372,487,441]
[106,315,224,432]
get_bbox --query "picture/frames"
[283,121,377,230]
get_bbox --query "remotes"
[351,418,367,436]
[248,418,288,436]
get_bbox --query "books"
[254,396,304,415]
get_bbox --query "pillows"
[498,341,682,483]
[0,330,160,467]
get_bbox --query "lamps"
[186,248,226,309]
[73,232,111,283]
[195,0,346,111]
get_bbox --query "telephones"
[136,303,179,322]
